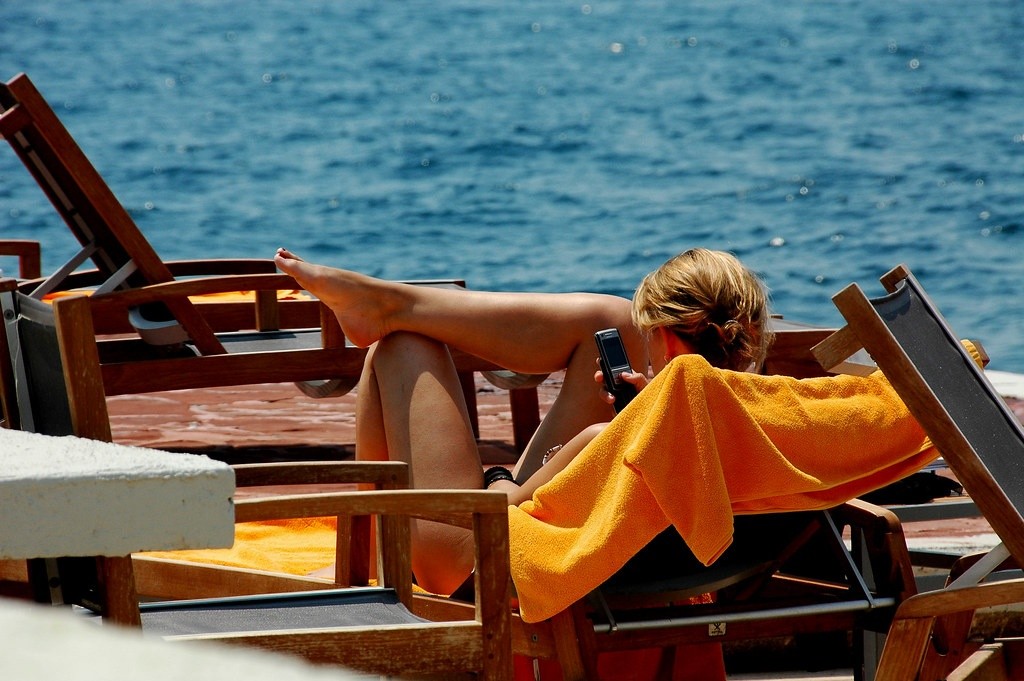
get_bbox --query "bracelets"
[483,464,520,489]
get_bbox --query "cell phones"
[595,328,637,415]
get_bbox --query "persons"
[273,248,769,601]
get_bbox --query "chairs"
[0,73,1024,681]
